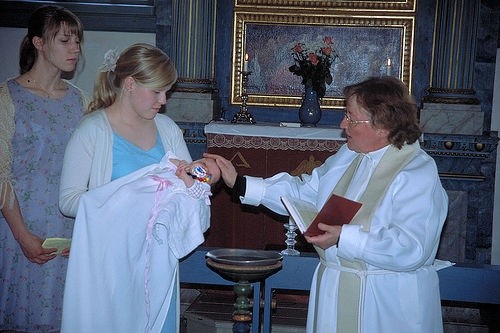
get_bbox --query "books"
[280,193,363,237]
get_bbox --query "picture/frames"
[234,0,418,16]
[230,10,416,110]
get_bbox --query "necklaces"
[26,71,61,99]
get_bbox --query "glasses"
[345,111,375,128]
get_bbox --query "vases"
[298,90,322,128]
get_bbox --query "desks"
[184,120,362,302]
[178,249,500,333]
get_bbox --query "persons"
[0,6,95,333]
[202,76,449,333]
[59,43,221,333]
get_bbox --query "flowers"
[289,37,337,88]
